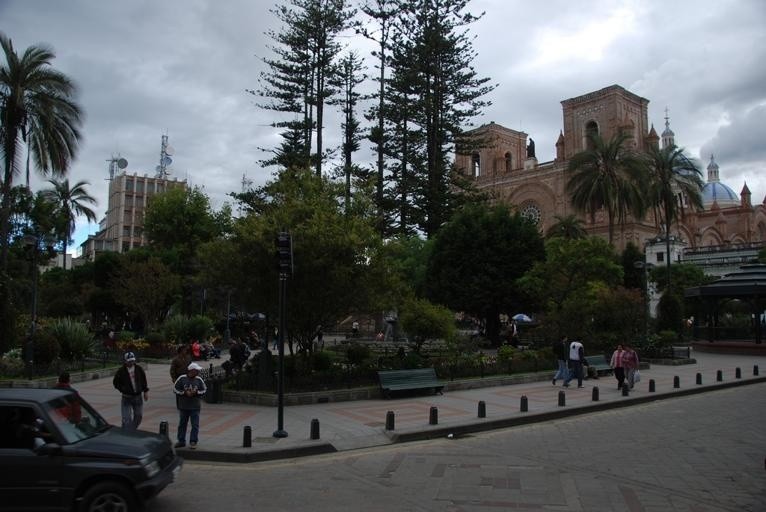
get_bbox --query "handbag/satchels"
[564,360,590,377]
[633,372,640,383]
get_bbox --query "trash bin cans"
[204,376,223,405]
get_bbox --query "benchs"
[584,354,613,379]
[671,345,695,359]
[376,367,444,400]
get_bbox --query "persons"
[508,320,520,337]
[609,344,626,389]
[48,373,81,427]
[192,326,280,376]
[553,335,569,385]
[376,331,384,341]
[173,363,207,449]
[352,319,359,337]
[14,407,52,448]
[499,310,509,329]
[170,346,191,410]
[113,352,148,430]
[622,344,640,389]
[563,338,589,388]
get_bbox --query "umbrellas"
[512,314,532,322]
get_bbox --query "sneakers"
[552,378,634,392]
[173,440,199,449]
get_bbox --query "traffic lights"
[273,233,288,272]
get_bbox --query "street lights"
[22,228,57,368]
[633,259,657,339]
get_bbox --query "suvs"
[0,385,186,511]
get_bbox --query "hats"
[187,361,202,372]
[122,351,136,363]
